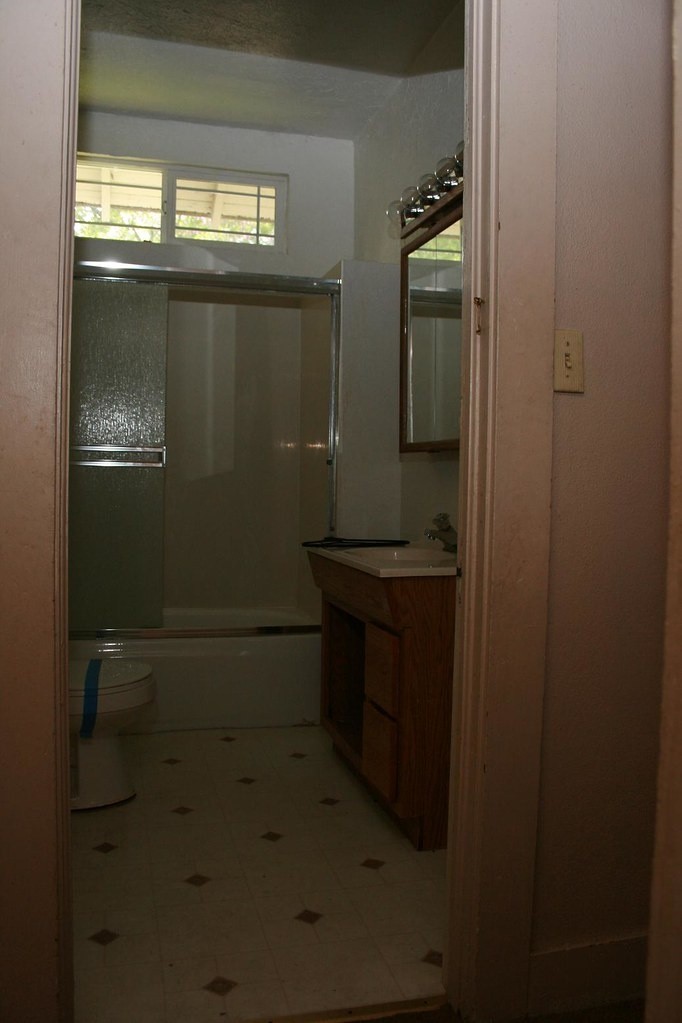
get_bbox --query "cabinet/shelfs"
[308,554,453,852]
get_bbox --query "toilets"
[68,654,155,812]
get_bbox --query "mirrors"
[399,206,463,452]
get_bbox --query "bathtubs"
[68,606,320,733]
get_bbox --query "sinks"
[339,542,457,566]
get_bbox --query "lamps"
[386,141,463,223]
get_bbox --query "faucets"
[424,511,458,550]
